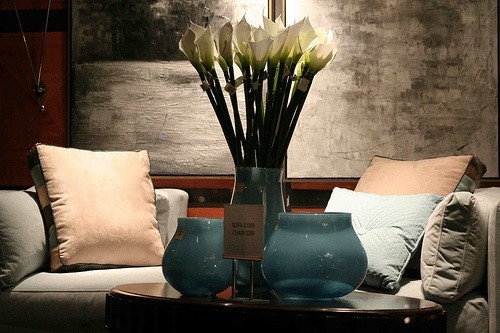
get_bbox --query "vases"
[161,167,368,302]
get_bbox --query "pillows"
[323,153,490,302]
[0,142,168,288]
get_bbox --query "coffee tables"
[105,281,446,333]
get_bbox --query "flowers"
[176,9,340,167]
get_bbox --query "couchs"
[0,175,499,333]
